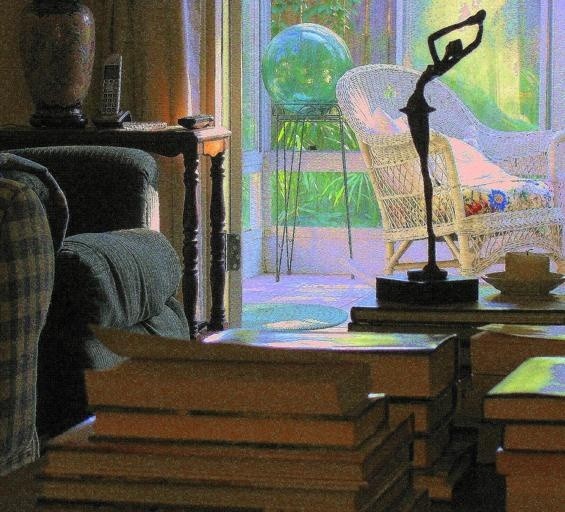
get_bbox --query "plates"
[481,272,565,295]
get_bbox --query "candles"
[504,250,550,278]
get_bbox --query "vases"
[21,2,98,129]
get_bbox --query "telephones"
[92,54,132,128]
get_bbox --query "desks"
[1,123,233,342]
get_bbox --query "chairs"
[0,145,192,477]
[336,64,565,274]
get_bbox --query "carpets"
[241,301,349,332]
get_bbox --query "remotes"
[177,114,215,130]
